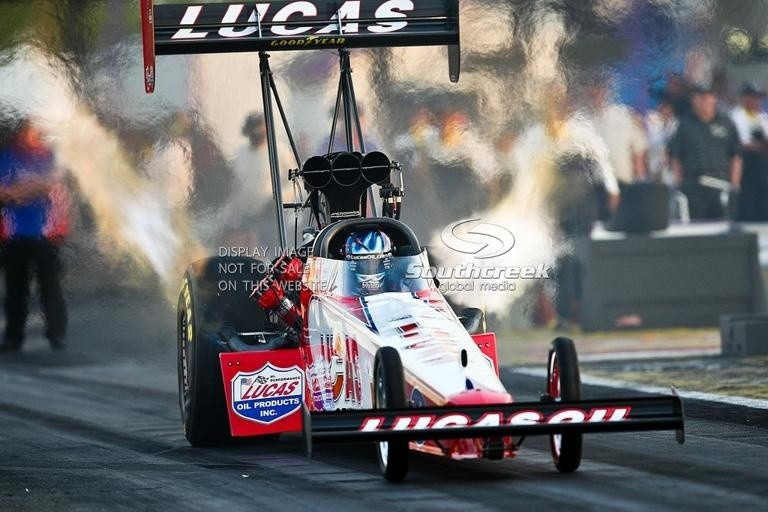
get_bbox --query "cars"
[138,1,685,482]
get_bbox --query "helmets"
[343,231,396,296]
